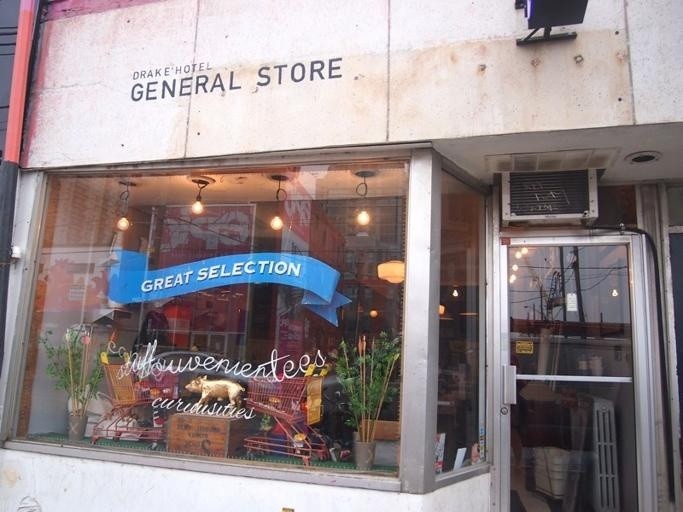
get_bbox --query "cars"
[298,337,398,417]
[142,348,284,396]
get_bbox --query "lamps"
[355,171,376,225]
[116,182,137,230]
[269,175,289,231]
[191,180,209,215]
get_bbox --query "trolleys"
[86,358,175,453]
[241,372,330,467]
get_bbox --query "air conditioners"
[501,167,599,227]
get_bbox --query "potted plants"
[35,323,118,442]
[334,331,402,471]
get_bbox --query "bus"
[271,269,402,380]
[32,244,249,363]
[509,334,634,397]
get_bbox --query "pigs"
[185,375,245,410]
